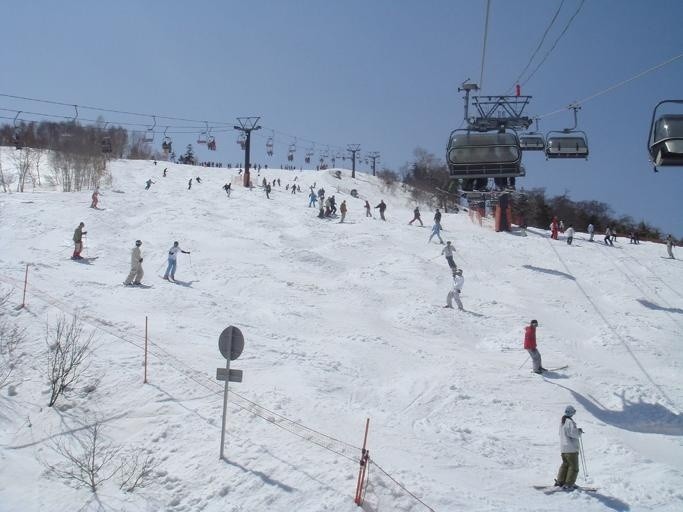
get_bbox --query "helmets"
[565,406,576,417]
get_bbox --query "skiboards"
[530,365,567,373]
[533,483,601,494]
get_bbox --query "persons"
[163,241,191,281]
[143,141,387,221]
[547,214,639,247]
[407,206,465,312]
[554,405,585,490]
[123,240,144,285]
[663,233,676,259]
[525,320,547,374]
[90,186,100,208]
[72,223,86,259]
